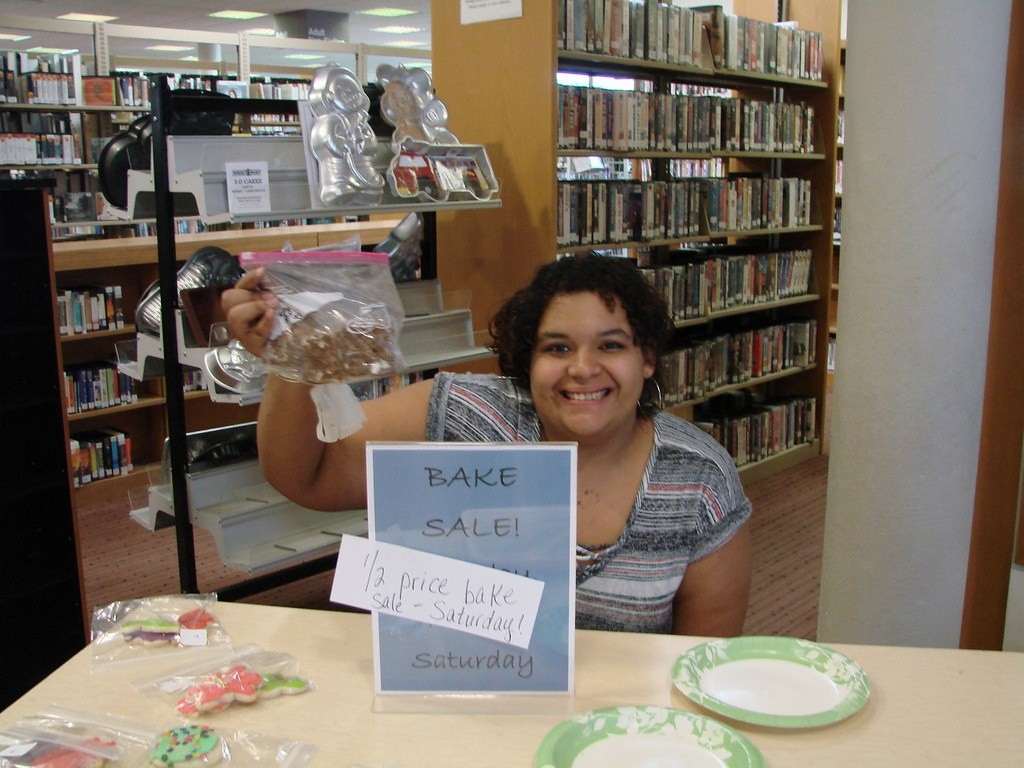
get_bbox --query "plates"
[671,633,871,731]
[530,703,766,768]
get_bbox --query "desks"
[1,593,1023,767]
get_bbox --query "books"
[0,51,361,488]
[693,384,816,467]
[559,83,816,154]
[637,250,813,321]
[557,0,825,80]
[656,320,818,402]
[556,159,811,249]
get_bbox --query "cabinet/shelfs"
[1,1,845,601]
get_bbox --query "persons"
[222,255,753,637]
